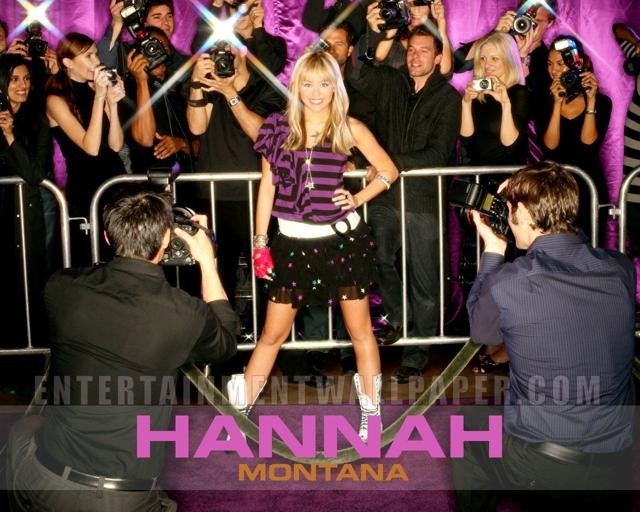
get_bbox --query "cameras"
[17,21,48,56]
[124,0,149,18]
[511,3,542,37]
[374,0,434,33]
[209,41,235,77]
[473,78,493,92]
[553,38,592,105]
[0,89,7,111]
[99,62,117,82]
[146,161,198,266]
[231,0,251,16]
[623,55,640,76]
[120,4,169,73]
[449,180,515,242]
[312,38,330,51]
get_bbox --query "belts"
[34,447,159,490]
[516,437,640,466]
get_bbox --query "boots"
[354,372,383,442]
[224,372,268,452]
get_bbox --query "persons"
[440,154,639,510]
[0,191,248,512]
[221,45,408,452]
[1,1,640,404]
